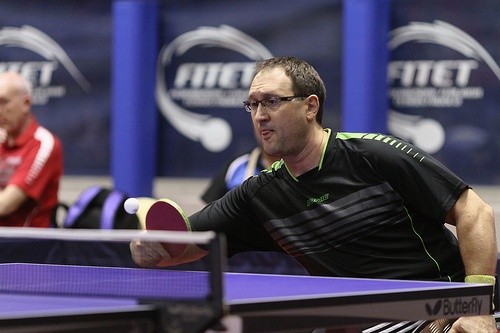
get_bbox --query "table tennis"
[123,197,140,215]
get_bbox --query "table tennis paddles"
[145,198,193,255]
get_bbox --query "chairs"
[96,191,131,267]
[42,186,105,263]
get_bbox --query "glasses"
[243,95,310,112]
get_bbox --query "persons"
[130,57,500,333]
[0,71,62,229]
